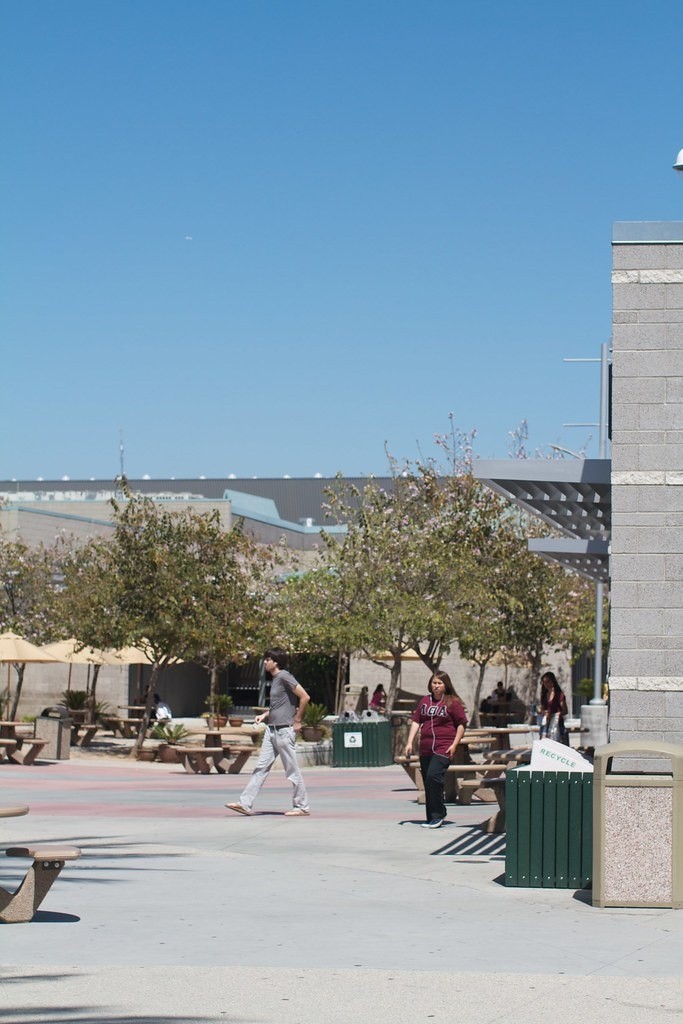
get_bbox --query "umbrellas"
[108,637,184,705]
[0,628,62,722]
[38,635,125,710]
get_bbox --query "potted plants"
[56,689,90,722]
[200,694,235,727]
[301,702,332,742]
[142,719,199,764]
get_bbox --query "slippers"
[284,809,310,815]
[224,803,250,816]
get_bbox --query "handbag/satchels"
[547,714,563,742]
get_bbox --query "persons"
[403,671,468,829]
[478,680,518,714]
[536,671,569,745]
[142,684,172,733]
[224,648,310,818]
[355,684,388,715]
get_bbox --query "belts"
[268,725,289,730]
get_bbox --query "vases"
[228,718,244,727]
[136,748,159,762]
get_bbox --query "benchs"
[23,738,51,765]
[100,714,171,739]
[81,724,104,747]
[393,744,595,835]
[178,744,257,775]
[0,738,18,761]
[0,844,82,924]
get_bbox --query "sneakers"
[420,813,447,828]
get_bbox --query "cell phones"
[406,752,410,759]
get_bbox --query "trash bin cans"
[343,685,362,712]
[333,710,392,766]
[505,762,593,889]
[592,741,683,909]
[33,706,71,759]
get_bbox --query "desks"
[383,697,417,710]
[187,728,261,775]
[0,801,30,818]
[117,704,158,738]
[467,728,530,765]
[531,727,589,748]
[417,737,498,804]
[464,732,490,738]
[50,709,89,745]
[0,721,35,765]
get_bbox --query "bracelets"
[294,718,302,724]
